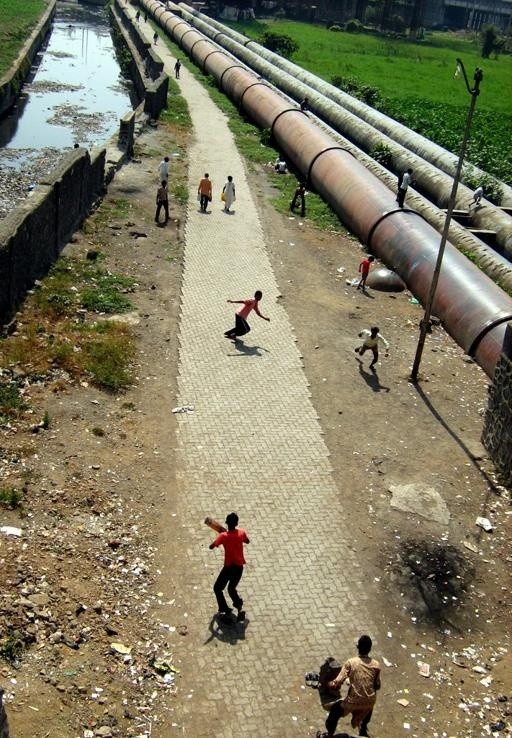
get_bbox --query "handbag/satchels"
[209,196,212,201]
[222,193,225,201]
[198,194,200,201]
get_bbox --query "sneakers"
[355,347,361,352]
[224,332,237,340]
[317,731,329,738]
[370,366,375,371]
[219,611,245,625]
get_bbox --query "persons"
[153,31,158,45]
[154,181,169,223]
[396,168,417,208]
[157,157,170,184]
[221,176,235,212]
[289,184,307,217]
[317,636,381,738]
[197,173,213,213]
[209,512,250,623]
[469,186,484,207]
[354,326,390,369]
[275,157,286,173]
[174,58,181,79]
[125,0,148,23]
[357,256,374,292]
[224,290,270,341]
[301,98,310,110]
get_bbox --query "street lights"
[410,58,483,382]
